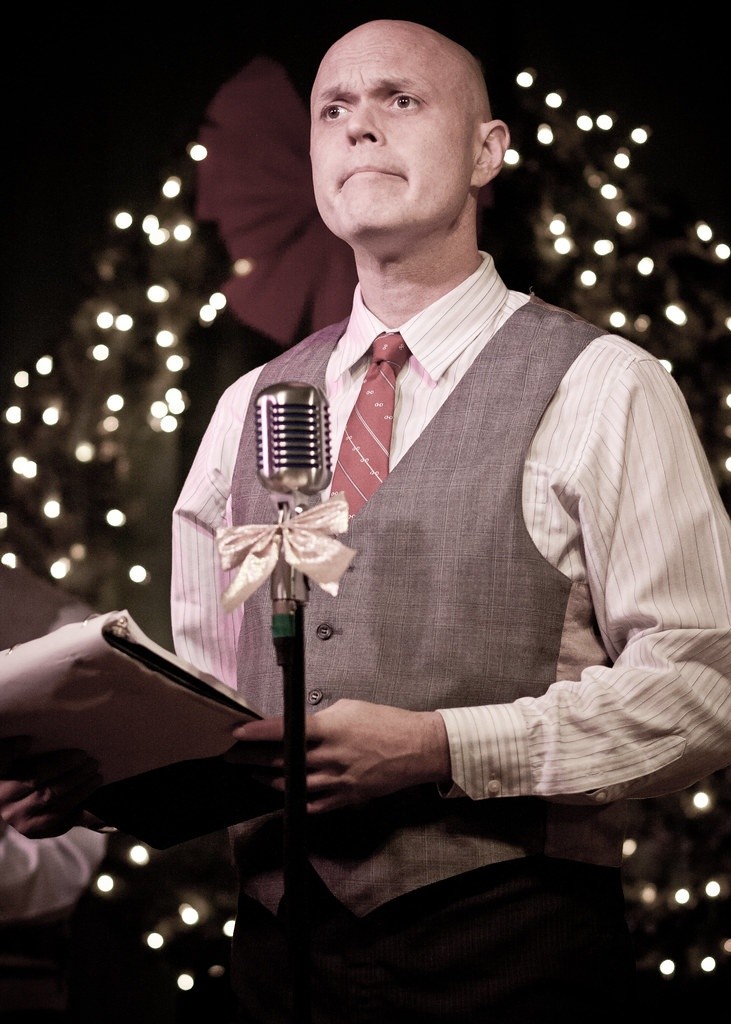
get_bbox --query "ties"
[328,331,413,540]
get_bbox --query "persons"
[1,18,729,1022]
